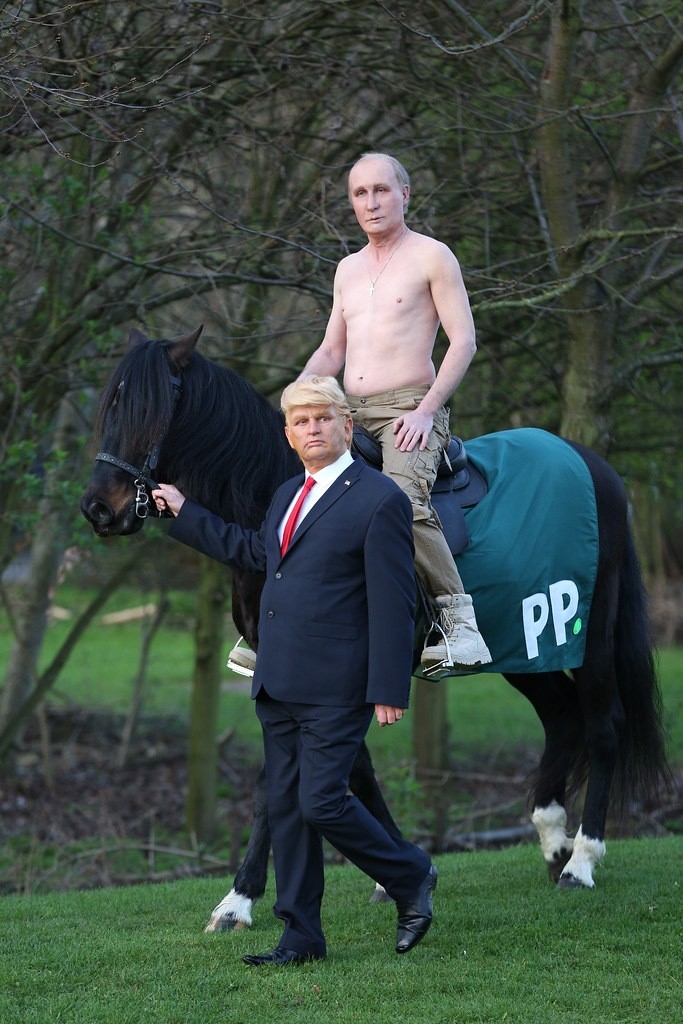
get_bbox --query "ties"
[281,477,316,558]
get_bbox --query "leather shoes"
[243,946,309,968]
[395,865,438,954]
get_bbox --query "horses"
[80,325,675,934]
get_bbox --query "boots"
[229,645,257,670]
[420,595,492,669]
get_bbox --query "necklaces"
[364,230,406,296]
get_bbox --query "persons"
[151,375,438,968]
[224,152,492,670]
